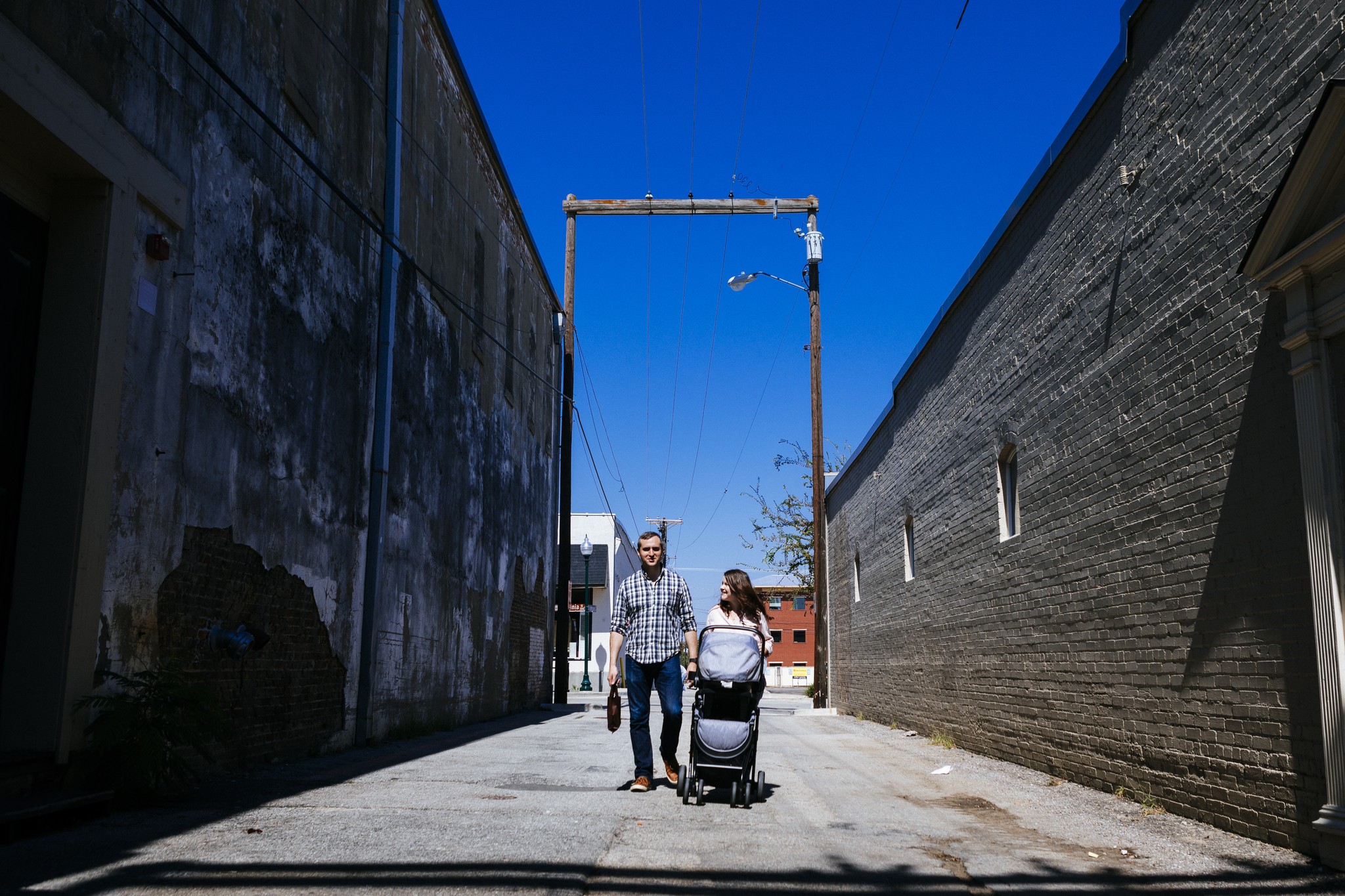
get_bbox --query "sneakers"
[660,751,680,784]
[630,776,652,792]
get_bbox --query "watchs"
[689,658,697,664]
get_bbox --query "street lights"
[729,271,845,704]
[581,534,595,690]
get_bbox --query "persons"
[680,665,687,691]
[609,531,698,792]
[703,569,774,659]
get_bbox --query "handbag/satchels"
[607,683,622,734]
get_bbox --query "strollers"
[679,625,767,810]
